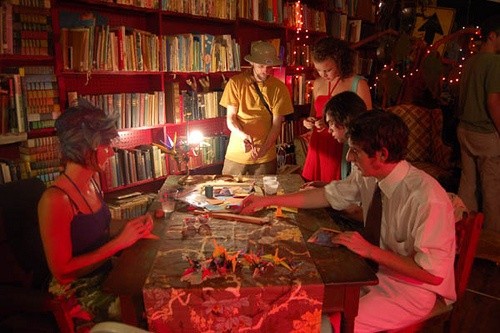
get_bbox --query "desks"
[105,173,379,333]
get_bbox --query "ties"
[364,182,382,274]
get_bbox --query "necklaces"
[63,171,111,236]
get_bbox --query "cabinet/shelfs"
[0,0,375,204]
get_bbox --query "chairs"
[427,211,484,333]
[0,177,59,333]
[386,105,454,182]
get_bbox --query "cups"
[263,176,279,197]
[158,187,179,212]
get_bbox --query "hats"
[243,41,280,65]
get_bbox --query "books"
[107,0,361,45]
[0,64,63,188]
[60,25,242,72]
[68,90,165,129]
[0,0,52,58]
[274,119,293,143]
[273,145,294,173]
[165,80,224,123]
[104,192,158,218]
[268,35,313,65]
[99,133,228,187]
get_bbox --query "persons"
[218,40,294,176]
[457,14,500,232]
[302,37,372,187]
[37,101,162,321]
[300,91,370,237]
[241,107,457,333]
